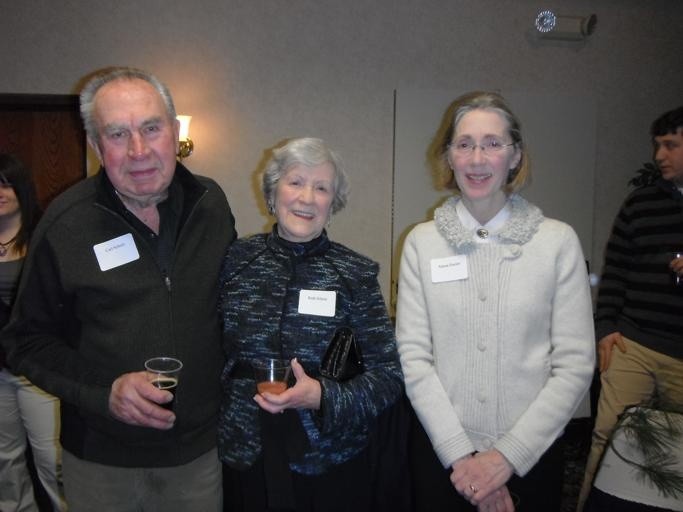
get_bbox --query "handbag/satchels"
[318,326,366,383]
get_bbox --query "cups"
[250,359,290,395]
[664,251,683,285]
[146,358,183,406]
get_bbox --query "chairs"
[584,406,683,511]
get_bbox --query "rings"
[468,483,479,493]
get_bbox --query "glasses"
[446,140,516,155]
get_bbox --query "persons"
[0,68,238,510]
[0,150,60,511]
[394,90,595,510]
[215,134,405,510]
[581,108,682,510]
